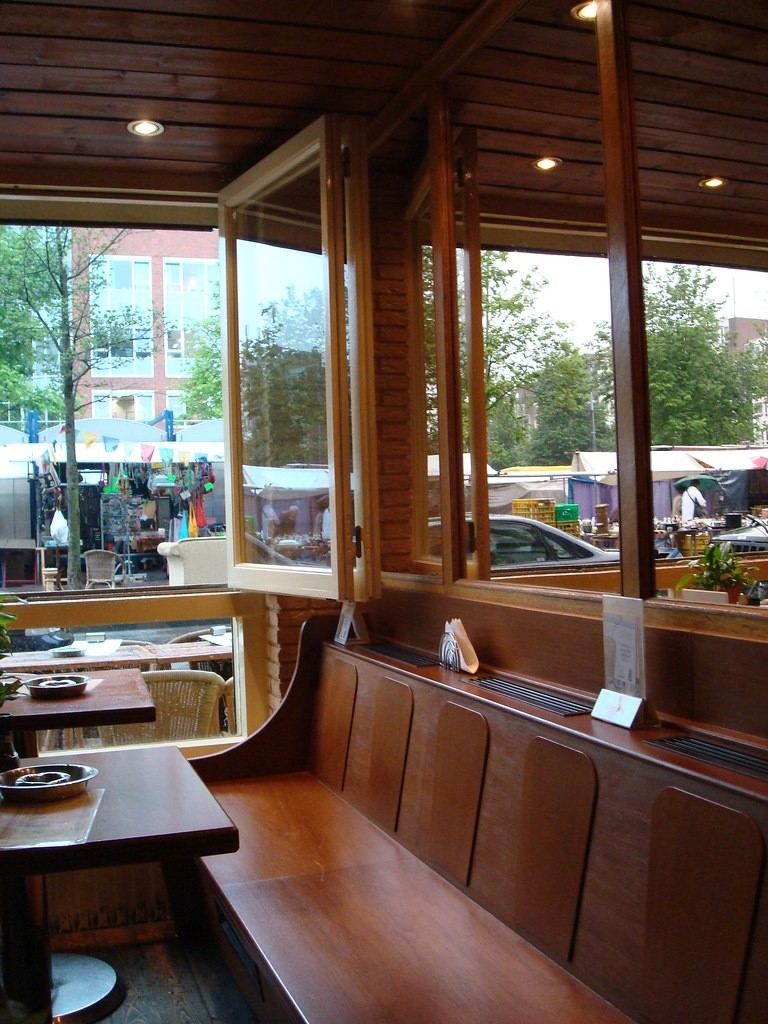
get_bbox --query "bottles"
[664,515,683,527]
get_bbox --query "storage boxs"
[557,522,580,537]
[245,515,254,533]
[555,504,578,521]
[544,523,556,528]
[681,549,705,558]
[676,535,710,550]
[512,498,555,523]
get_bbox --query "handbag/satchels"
[694,498,707,517]
[50,510,68,546]
[97,461,216,540]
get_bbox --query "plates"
[23,675,91,700]
[0,763,99,802]
[50,648,85,658]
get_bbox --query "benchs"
[171,613,768,1024]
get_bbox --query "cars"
[701,514,768,554]
[428,510,620,569]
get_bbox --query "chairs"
[83,549,128,590]
[582,517,726,556]
[164,626,235,680]
[98,670,223,748]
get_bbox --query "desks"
[148,643,233,681]
[0,745,241,1024]
[0,668,158,1024]
[0,644,155,674]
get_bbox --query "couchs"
[156,536,233,586]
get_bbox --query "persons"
[672,480,706,520]
[273,495,329,539]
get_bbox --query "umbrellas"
[674,475,719,492]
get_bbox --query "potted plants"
[677,540,757,605]
